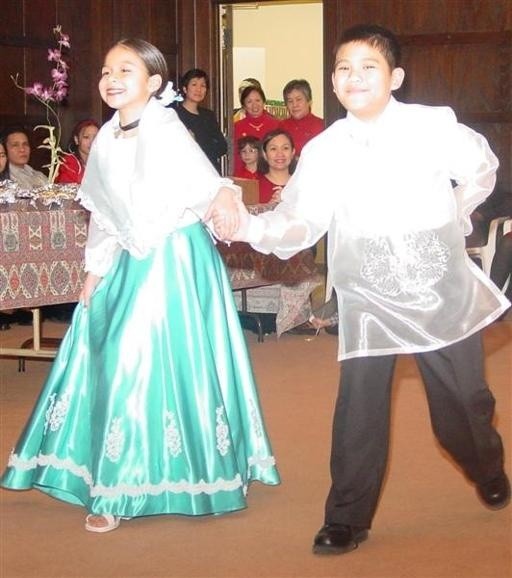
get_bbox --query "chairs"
[467,215,512,319]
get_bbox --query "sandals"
[83,512,123,533]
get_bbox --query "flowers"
[8,24,74,187]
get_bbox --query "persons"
[0,39,281,535]
[230,77,264,121]
[4,126,51,188]
[55,119,102,183]
[213,23,510,557]
[253,128,304,205]
[235,135,267,181]
[0,143,7,181]
[233,84,283,180]
[173,68,228,174]
[278,80,325,154]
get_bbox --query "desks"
[0,197,317,373]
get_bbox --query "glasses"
[239,148,258,155]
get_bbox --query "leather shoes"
[0,311,19,325]
[476,470,511,512]
[13,309,34,323]
[311,521,370,556]
